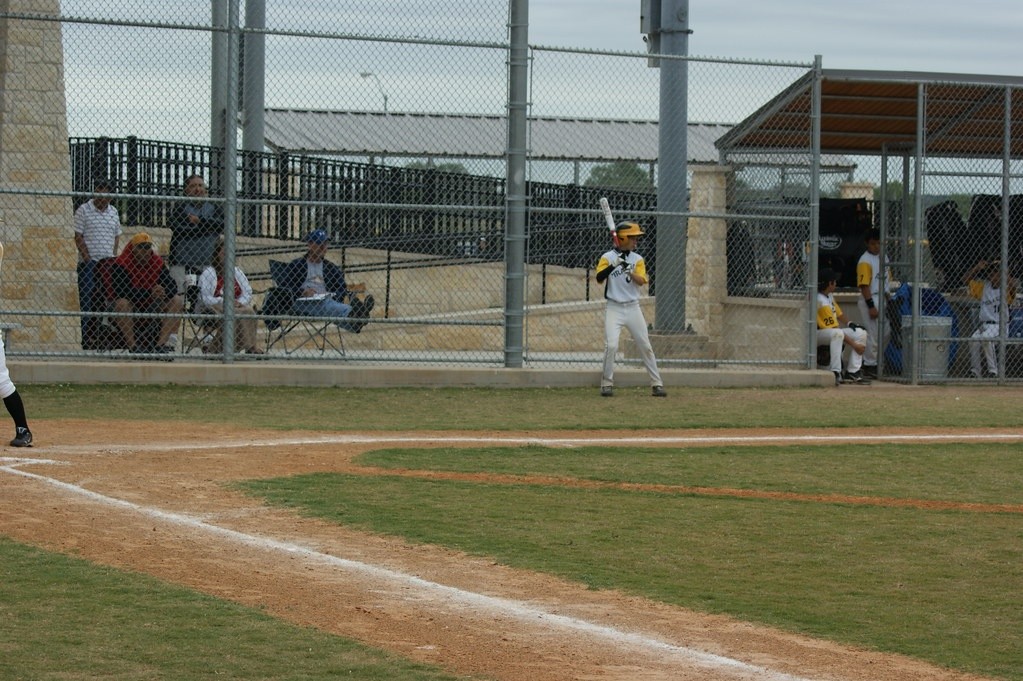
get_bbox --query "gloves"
[611,252,625,268]
[621,261,633,276]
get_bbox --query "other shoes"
[350,298,362,334]
[130,347,150,362]
[967,372,977,378]
[246,348,272,361]
[861,362,887,377]
[154,346,174,361]
[364,295,374,325]
[984,372,997,378]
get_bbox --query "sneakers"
[11,427,34,446]
[844,371,871,385]
[834,371,844,384]
[601,385,613,396]
[653,385,667,395]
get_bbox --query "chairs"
[264,259,346,358]
[179,264,224,354]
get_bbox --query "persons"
[0,241,31,447]
[962,261,1015,378]
[817,268,871,384]
[597,221,668,397]
[110,231,181,361]
[283,230,374,332]
[856,229,893,378]
[195,239,268,361]
[166,174,224,352]
[75,183,122,347]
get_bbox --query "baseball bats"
[599,197,629,274]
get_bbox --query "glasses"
[136,243,150,249]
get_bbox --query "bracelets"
[847,321,852,327]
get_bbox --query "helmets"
[616,221,644,247]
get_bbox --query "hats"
[818,269,842,285]
[134,233,152,244]
[307,230,327,244]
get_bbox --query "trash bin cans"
[902,315,953,382]
